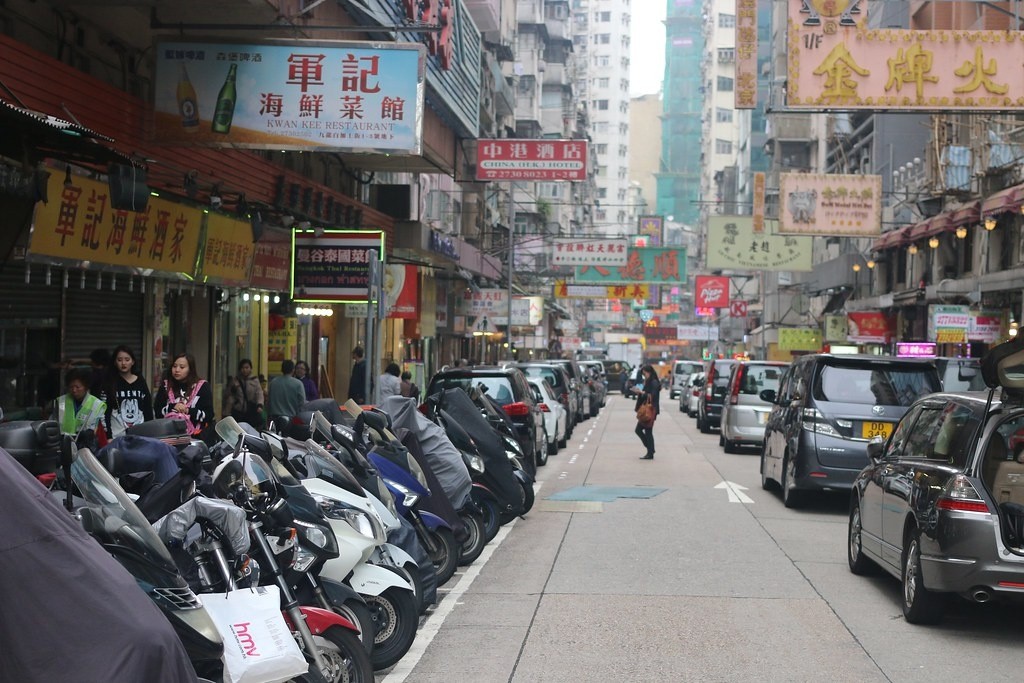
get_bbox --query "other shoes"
[647,449,655,454]
[640,452,653,459]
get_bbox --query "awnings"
[873,184,1024,252]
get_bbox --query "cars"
[847,330,1023,625]
[679,372,705,417]
[499,359,653,457]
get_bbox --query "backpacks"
[164,379,207,435]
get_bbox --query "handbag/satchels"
[637,393,656,428]
[197,567,309,683]
[247,404,262,427]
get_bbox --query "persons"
[455,359,477,367]
[46,375,107,475]
[348,347,374,405]
[372,363,401,406]
[294,361,319,403]
[400,372,418,406]
[268,360,305,435]
[90,349,114,402]
[619,365,645,400]
[154,353,214,442]
[630,365,661,459]
[106,345,154,444]
[223,359,264,433]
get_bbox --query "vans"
[760,353,946,514]
[668,359,706,399]
[718,360,792,453]
[693,359,737,435]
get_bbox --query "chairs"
[982,431,1024,509]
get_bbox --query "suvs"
[425,361,549,468]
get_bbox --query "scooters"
[1,375,537,683]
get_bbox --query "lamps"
[956,224,968,239]
[928,235,939,249]
[867,258,892,268]
[109,160,150,213]
[249,206,269,242]
[852,262,865,272]
[907,242,918,255]
[983,214,1001,231]
[915,195,943,219]
